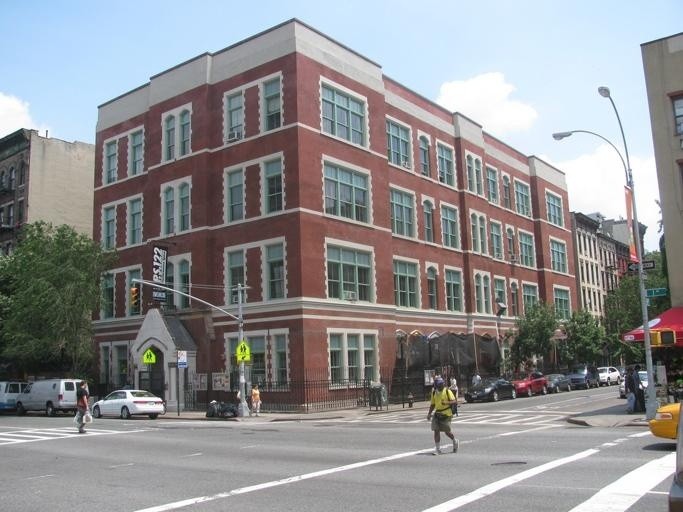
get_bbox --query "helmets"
[435,377,445,385]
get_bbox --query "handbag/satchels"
[83,413,93,424]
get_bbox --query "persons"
[77,381,89,433]
[249,384,261,416]
[496,362,536,380]
[471,373,481,386]
[431,371,458,401]
[427,377,460,455]
[633,365,647,412]
[624,366,639,411]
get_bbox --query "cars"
[619,370,648,397]
[649,400,683,512]
[91,390,166,420]
[464,365,620,403]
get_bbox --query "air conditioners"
[233,295,238,302]
[226,130,240,143]
[511,254,518,262]
[493,198,497,203]
[404,162,413,169]
[344,290,357,300]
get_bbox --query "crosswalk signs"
[142,348,156,363]
[235,340,251,362]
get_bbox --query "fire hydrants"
[408,392,413,407]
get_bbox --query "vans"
[0,378,89,417]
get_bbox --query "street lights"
[552,86,656,419]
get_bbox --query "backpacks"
[451,404,458,415]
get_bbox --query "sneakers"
[453,439,459,453]
[432,449,442,455]
[251,413,260,417]
[79,429,87,433]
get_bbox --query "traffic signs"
[628,261,655,271]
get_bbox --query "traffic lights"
[131,288,138,305]
